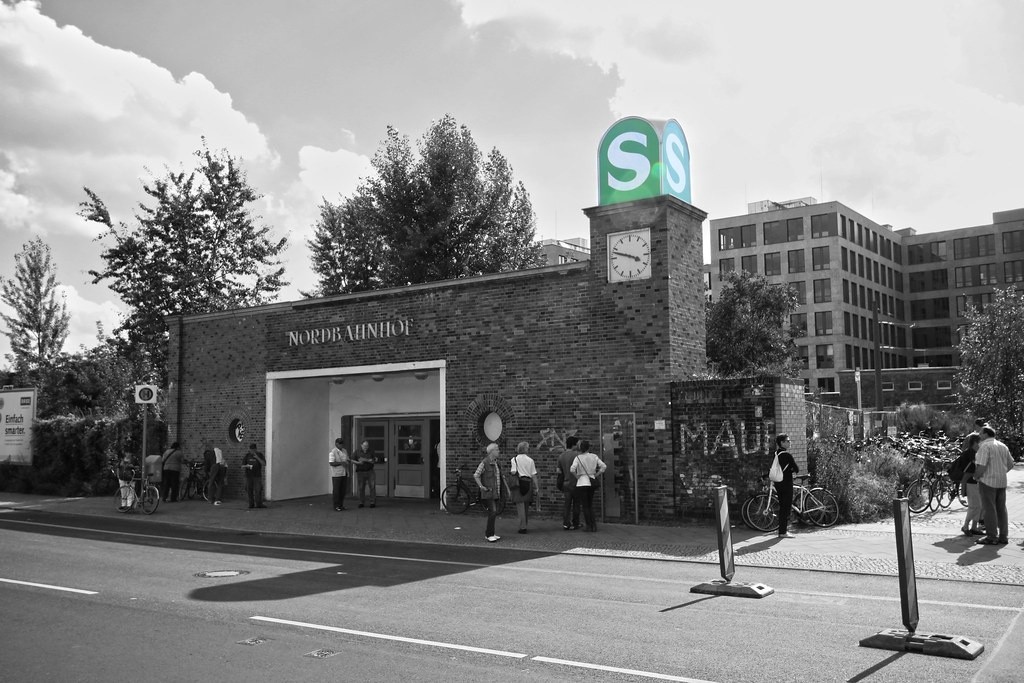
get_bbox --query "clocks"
[607,227,652,283]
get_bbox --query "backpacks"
[769,451,789,482]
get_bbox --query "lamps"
[371,374,385,382]
[331,376,345,384]
[414,371,428,380]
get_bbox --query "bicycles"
[739,470,840,533]
[838,427,970,514]
[112,468,160,515]
[440,467,506,515]
[176,458,219,502]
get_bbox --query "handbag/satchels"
[507,456,519,487]
[948,464,964,482]
[590,477,601,487]
[119,469,136,481]
[556,472,564,490]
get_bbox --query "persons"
[350,440,380,508]
[117,447,141,513]
[328,438,349,512]
[961,425,1015,545]
[769,433,799,538]
[241,444,268,508]
[203,447,229,506]
[509,441,540,535]
[434,442,440,502]
[557,437,607,532]
[473,443,512,543]
[160,442,188,504]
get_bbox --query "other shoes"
[359,504,364,508]
[979,523,987,530]
[518,529,528,534]
[571,523,583,529]
[961,526,973,537]
[779,531,794,538]
[970,528,985,536]
[335,507,342,512]
[249,505,255,508]
[977,537,999,545]
[493,534,500,539]
[485,535,497,542]
[370,504,376,508]
[1000,537,1008,545]
[564,523,571,529]
[256,504,266,508]
[341,506,348,510]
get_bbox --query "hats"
[335,438,344,444]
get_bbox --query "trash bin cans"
[145,455,162,483]
